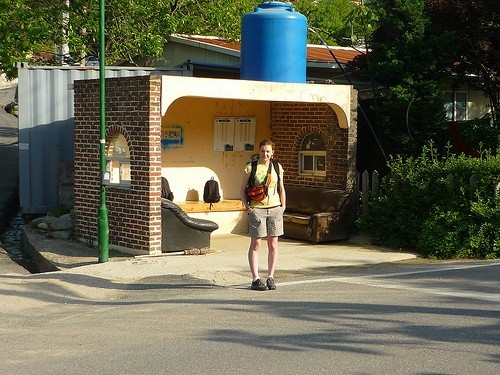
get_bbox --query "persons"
[240,140,286,291]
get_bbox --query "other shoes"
[250,279,268,291]
[266,277,276,290]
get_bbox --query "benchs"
[174,198,249,233]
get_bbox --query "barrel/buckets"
[240,2,308,83]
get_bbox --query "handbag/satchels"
[246,160,272,202]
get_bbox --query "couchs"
[161,197,219,251]
[281,186,352,242]
